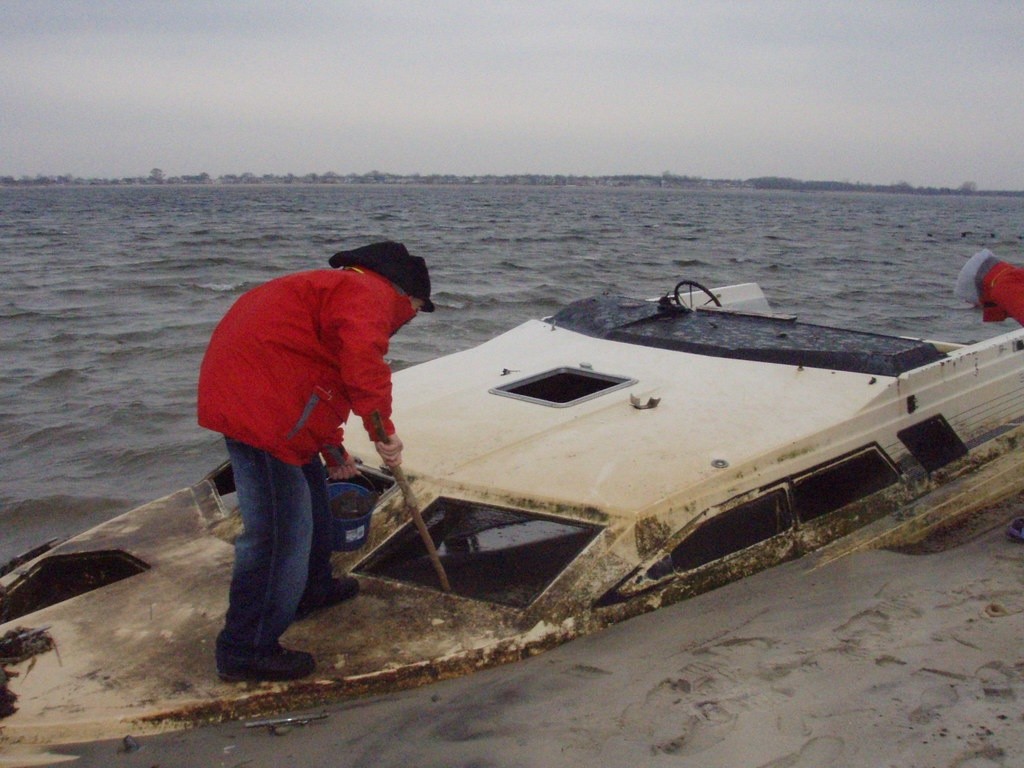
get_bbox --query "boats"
[0,277,1024,749]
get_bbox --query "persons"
[956,249,1024,542]
[197,240,436,685]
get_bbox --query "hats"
[329,240,435,313]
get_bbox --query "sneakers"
[216,644,315,682]
[291,576,359,622]
